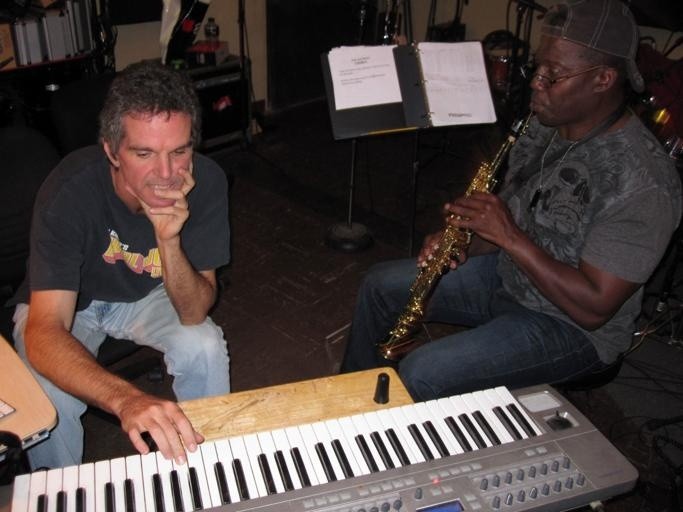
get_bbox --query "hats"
[539,0,646,93]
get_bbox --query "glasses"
[519,64,607,89]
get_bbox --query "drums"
[640,95,683,168]
[483,55,523,91]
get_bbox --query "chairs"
[48,79,233,291]
[551,288,683,401]
[0,128,162,428]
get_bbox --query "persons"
[337,1,683,408]
[0,55,232,476]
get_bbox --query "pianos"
[1,384,640,512]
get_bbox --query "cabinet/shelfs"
[183,56,251,149]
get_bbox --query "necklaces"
[526,129,579,214]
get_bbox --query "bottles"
[655,292,668,318]
[204,18,219,67]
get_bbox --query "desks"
[1,335,58,465]
